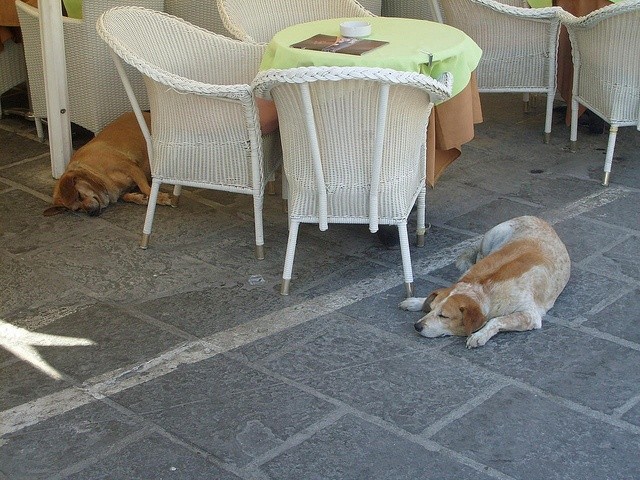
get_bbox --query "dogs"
[397,214,574,350]
[40,109,173,217]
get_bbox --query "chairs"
[382,0,564,145]
[15,0,165,142]
[562,0,640,187]
[95,5,282,260]
[217,0,379,44]
[252,66,454,297]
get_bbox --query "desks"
[258,17,483,191]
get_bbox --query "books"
[289,33,389,56]
